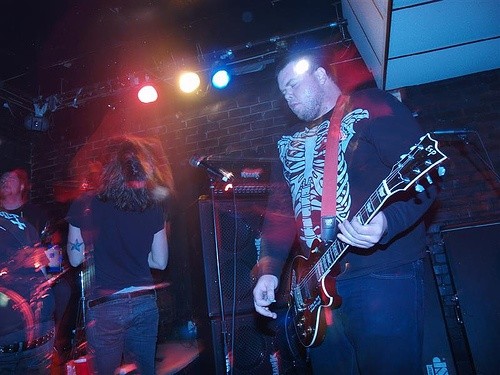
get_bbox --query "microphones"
[426,128,469,136]
[189,155,230,182]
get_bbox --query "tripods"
[60,248,94,365]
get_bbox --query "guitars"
[289,132,448,348]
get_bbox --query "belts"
[0,329,55,353]
[89,288,155,307]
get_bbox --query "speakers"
[198,194,290,318]
[210,307,313,375]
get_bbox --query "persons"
[0,165,45,239]
[66,134,171,374]
[39,175,78,341]
[250,43,440,374]
[0,212,58,375]
